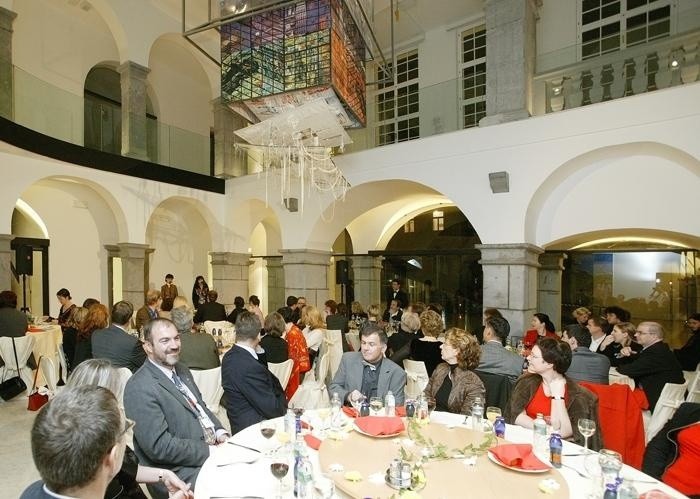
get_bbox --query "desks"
[189,409,692,499]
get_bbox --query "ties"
[172,373,181,389]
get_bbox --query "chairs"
[0,302,700,472]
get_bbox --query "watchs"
[548,396,566,400]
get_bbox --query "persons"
[421,327,486,415]
[124,317,229,493]
[56,288,76,362]
[21,385,135,498]
[1,291,38,370]
[641,401,700,496]
[278,280,443,360]
[63,274,289,374]
[329,326,407,407]
[503,338,603,452]
[412,306,699,414]
[68,359,193,497]
[221,311,288,436]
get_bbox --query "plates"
[352,420,401,438]
[488,451,551,473]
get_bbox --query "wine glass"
[486,407,502,424]
[598,448,623,488]
[350,314,367,331]
[351,391,365,417]
[578,418,596,453]
[369,396,384,416]
[311,477,336,499]
[270,456,288,498]
[386,319,399,333]
[293,400,306,416]
[544,416,561,433]
[260,418,275,450]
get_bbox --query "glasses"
[116,419,136,438]
[635,329,654,335]
[530,351,543,360]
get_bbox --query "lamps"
[219,1,370,224]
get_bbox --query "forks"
[462,415,469,425]
[216,458,260,467]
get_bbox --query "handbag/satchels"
[27,392,49,411]
[0,377,27,401]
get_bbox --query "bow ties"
[360,360,375,371]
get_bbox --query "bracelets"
[159,469,163,483]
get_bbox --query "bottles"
[361,403,370,416]
[298,453,314,499]
[517,339,525,350]
[472,397,484,428]
[212,328,216,335]
[293,457,299,496]
[218,340,222,347]
[296,417,302,436]
[549,433,562,467]
[603,483,617,498]
[283,409,295,433]
[385,390,395,416]
[218,329,222,335]
[533,413,546,442]
[620,475,640,499]
[494,415,506,439]
[330,392,341,423]
[405,403,415,418]
[504,341,512,351]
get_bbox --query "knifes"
[227,441,261,454]
[209,496,263,499]
